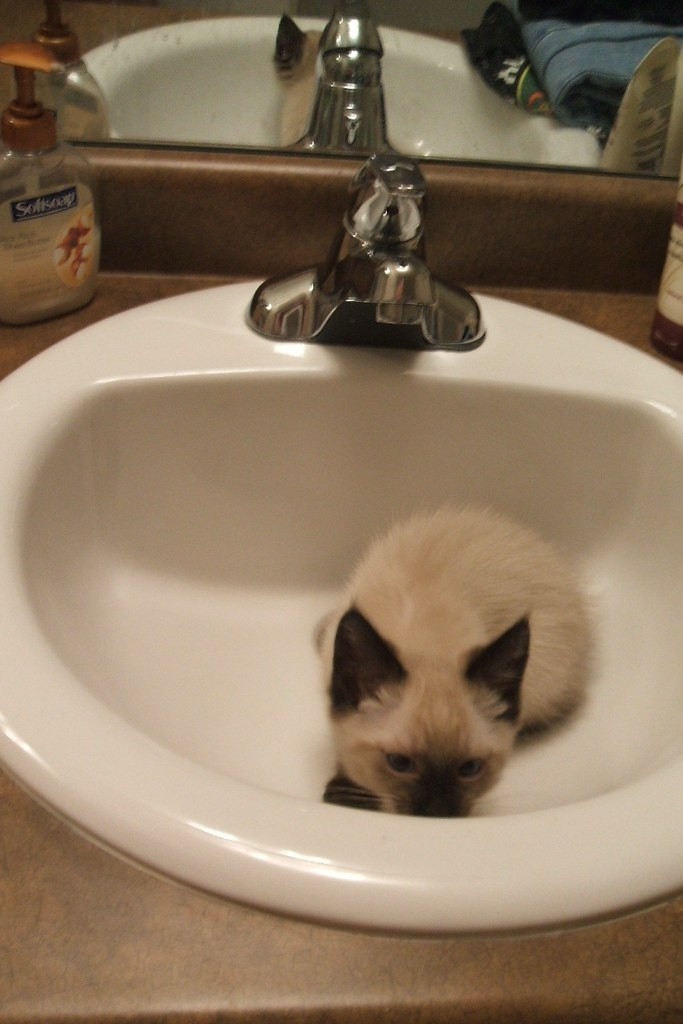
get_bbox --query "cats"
[321,502,600,819]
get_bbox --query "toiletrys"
[0,39,105,326]
[598,34,678,172]
[644,179,683,356]
[32,0,110,141]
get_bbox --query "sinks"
[2,281,683,935]
[47,12,603,170]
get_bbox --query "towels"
[458,1,680,144]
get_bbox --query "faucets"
[308,153,441,343]
[301,0,395,150]
